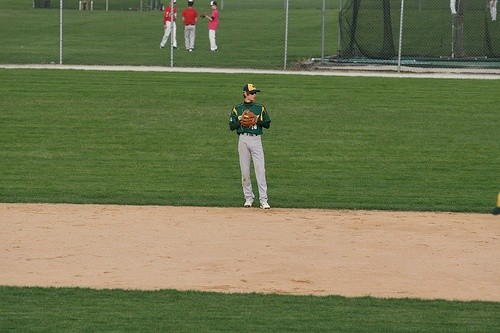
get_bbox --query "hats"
[244,84,260,93]
[208,1,217,6]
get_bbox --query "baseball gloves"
[238,109,257,127]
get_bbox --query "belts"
[239,133,258,136]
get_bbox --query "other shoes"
[244,199,254,207]
[259,201,271,209]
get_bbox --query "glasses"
[250,92,256,95]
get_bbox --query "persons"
[228,84,272,209]
[200,1,218,52]
[160,0,179,50]
[181,0,199,51]
